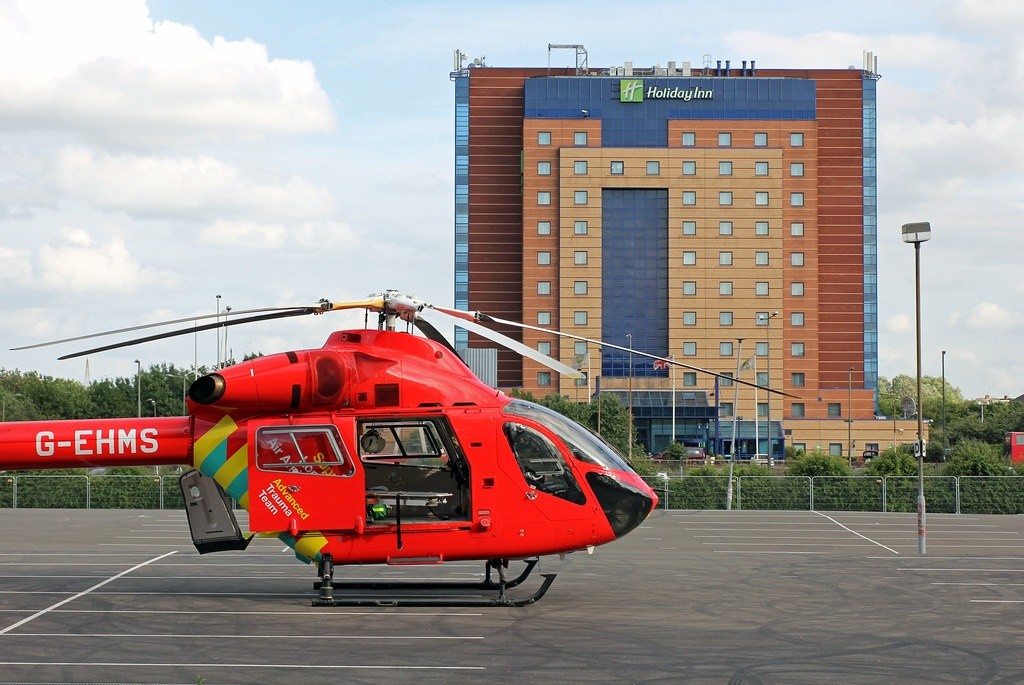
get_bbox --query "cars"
[748,453,776,467]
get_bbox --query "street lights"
[134,358,141,417]
[899,221,939,556]
[726,338,746,508]
[941,349,947,464]
[766,311,779,468]
[848,366,856,466]
[165,373,188,417]
[224,305,234,372]
[215,293,222,372]
[145,398,157,417]
[625,333,633,461]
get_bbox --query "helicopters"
[0,286,793,607]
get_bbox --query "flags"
[572,353,589,368]
[653,354,672,370]
[739,355,755,373]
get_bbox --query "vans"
[648,446,706,460]
